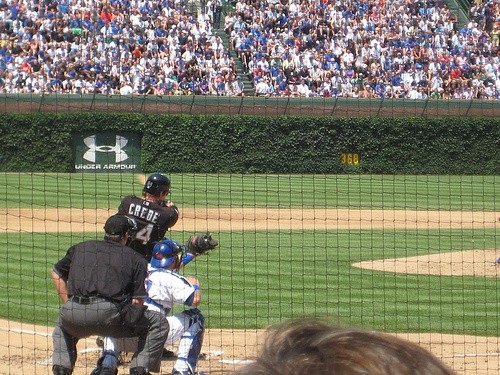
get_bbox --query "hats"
[103,215,133,236]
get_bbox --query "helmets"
[151,239,181,268]
[142,174,170,197]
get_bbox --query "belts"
[70,296,95,305]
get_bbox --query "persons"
[50,214,170,375]
[2,1,500,99]
[222,317,460,375]
[91,233,219,375]
[101,171,179,267]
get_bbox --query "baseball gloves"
[185,232,219,255]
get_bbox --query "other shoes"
[130,367,151,375]
[91,365,117,375]
[52,366,73,375]
[162,349,174,357]
[173,369,207,375]
